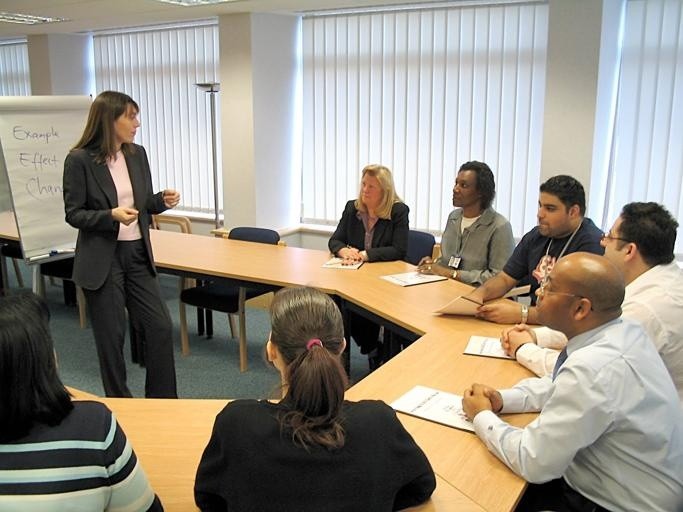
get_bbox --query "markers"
[29,249,75,262]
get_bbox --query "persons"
[329,164,410,372]
[63,91,182,398]
[194,286,436,512]
[500,202,683,402]
[468,175,605,326]
[462,251,683,512]
[0,287,165,512]
[415,161,515,288]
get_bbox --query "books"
[379,271,448,287]
[389,385,500,432]
[321,256,365,269]
[433,296,484,316]
[463,335,517,361]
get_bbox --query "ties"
[551,343,569,386]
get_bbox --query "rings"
[426,265,432,271]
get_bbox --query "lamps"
[193,83,219,237]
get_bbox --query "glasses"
[537,277,577,306]
[600,231,631,244]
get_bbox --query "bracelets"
[451,269,459,280]
[520,304,529,324]
[515,342,527,360]
